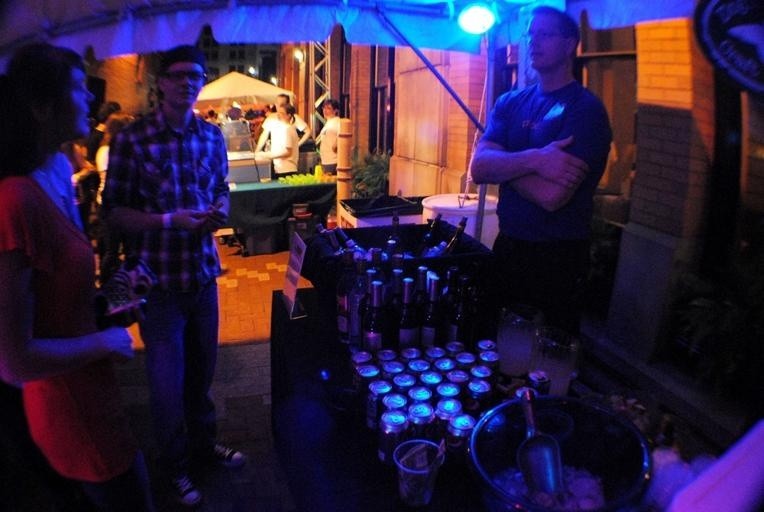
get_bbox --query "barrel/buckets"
[421,194,501,249]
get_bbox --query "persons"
[0,41,153,512]
[320,100,339,175]
[101,46,247,505]
[255,94,311,152]
[469,4,612,308]
[64,101,143,219]
[256,106,299,180]
[194,105,271,149]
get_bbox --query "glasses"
[160,70,208,81]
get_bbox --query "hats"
[160,46,210,75]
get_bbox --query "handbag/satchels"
[83,216,107,241]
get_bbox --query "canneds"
[515,370,550,399]
[350,340,500,467]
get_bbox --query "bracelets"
[161,213,172,230]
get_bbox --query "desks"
[229,179,338,257]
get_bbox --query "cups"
[391,438,446,505]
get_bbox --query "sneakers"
[172,474,202,505]
[210,444,244,466]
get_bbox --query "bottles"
[337,253,356,343]
[386,209,406,247]
[412,266,428,302]
[438,216,468,249]
[348,259,368,345]
[388,269,402,344]
[518,389,563,498]
[390,289,400,307]
[359,269,377,346]
[444,288,459,320]
[361,281,392,351]
[417,213,443,254]
[424,236,469,255]
[442,266,460,305]
[315,222,367,257]
[372,248,386,283]
[419,278,443,344]
[389,253,406,279]
[397,277,422,346]
[448,275,471,339]
